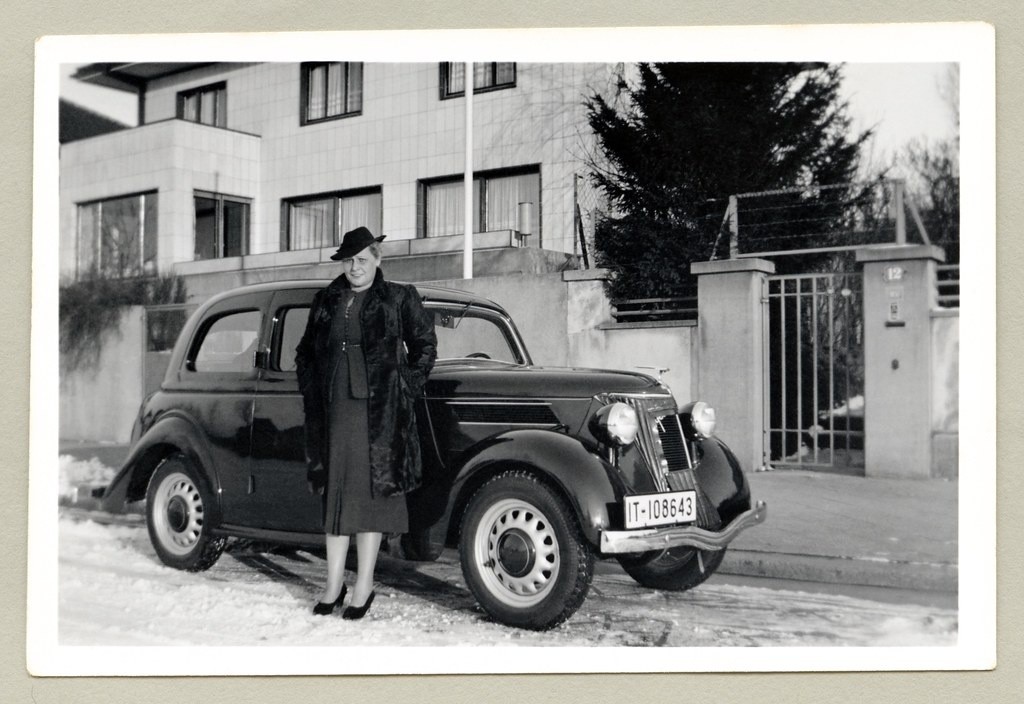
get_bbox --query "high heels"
[342,590,376,620]
[313,582,347,615]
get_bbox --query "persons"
[294,226,437,621]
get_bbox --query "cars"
[91,276,769,633]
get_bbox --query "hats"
[329,227,387,261]
